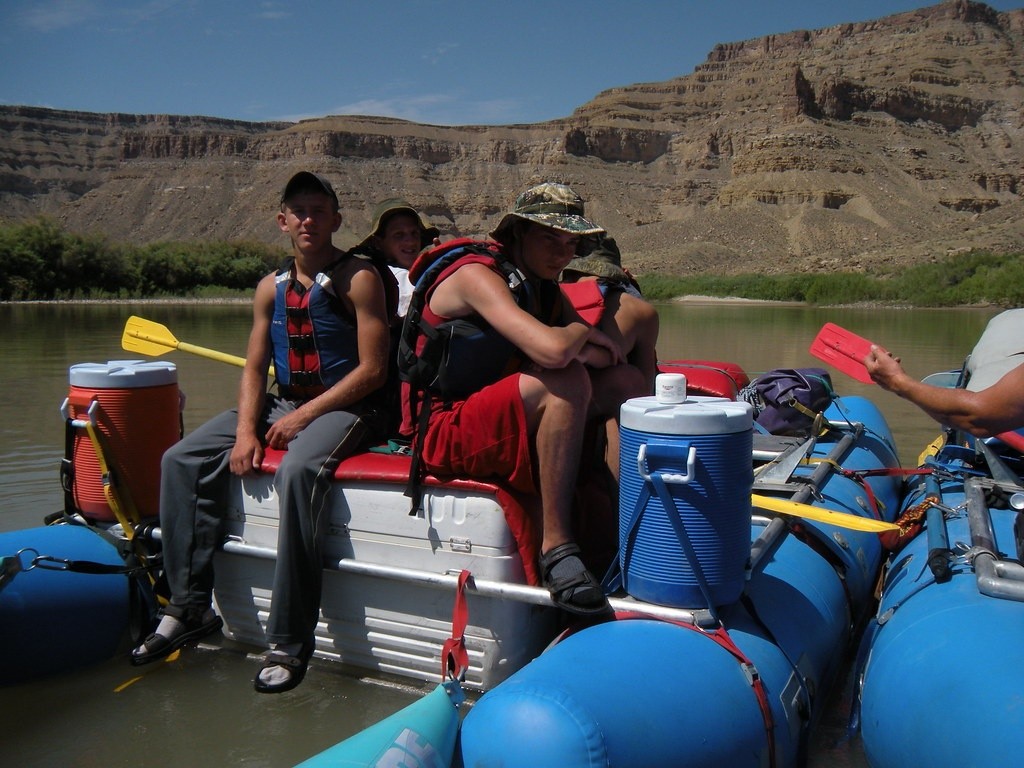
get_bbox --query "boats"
[1,358,1024,768]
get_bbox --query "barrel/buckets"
[619,396,752,609]
[60,359,185,522]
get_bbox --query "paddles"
[750,490,902,536]
[115,311,273,376]
[807,323,1023,453]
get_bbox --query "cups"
[655,373,687,403]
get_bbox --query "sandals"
[130,603,223,666]
[537,542,615,618]
[254,632,316,694]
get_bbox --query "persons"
[863,344,1024,438]
[132,171,401,693]
[344,196,657,515]
[398,182,648,615]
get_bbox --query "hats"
[490,184,609,248]
[348,198,441,251]
[558,238,631,283]
[279,170,339,213]
